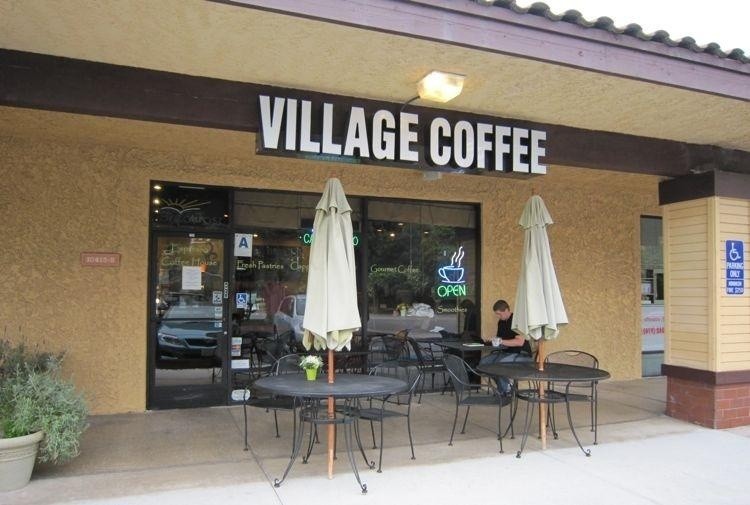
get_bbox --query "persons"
[479,300,534,397]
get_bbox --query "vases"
[306,370,316,381]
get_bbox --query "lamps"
[401,70,467,114]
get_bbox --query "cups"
[492,337,502,346]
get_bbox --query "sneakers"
[505,386,517,404]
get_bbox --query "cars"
[157,301,222,368]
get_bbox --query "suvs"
[272,294,307,354]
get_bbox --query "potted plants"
[0,339,90,492]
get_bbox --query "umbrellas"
[510,187,568,450]
[300,171,360,480]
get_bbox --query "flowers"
[300,355,324,370]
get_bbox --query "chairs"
[275,355,322,447]
[543,350,600,440]
[407,336,449,393]
[353,360,421,472]
[442,353,507,452]
